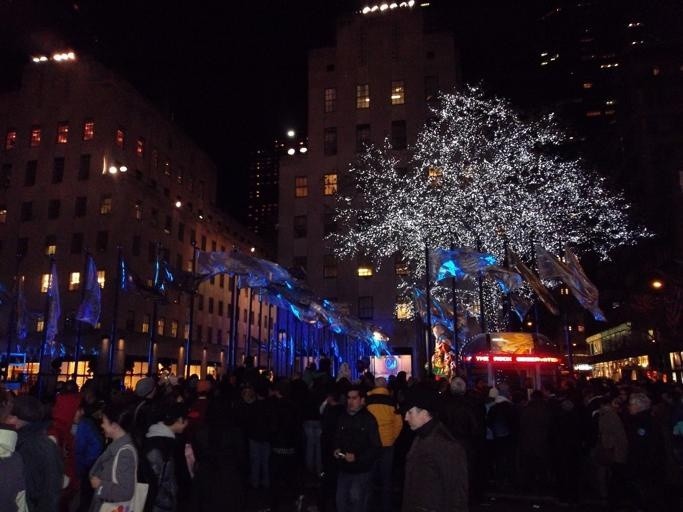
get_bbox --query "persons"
[1,356,683,512]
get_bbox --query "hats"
[167,402,199,418]
[395,386,433,415]
[136,373,161,398]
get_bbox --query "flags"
[416,248,607,331]
[0,251,393,357]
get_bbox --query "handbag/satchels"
[152,460,178,510]
[99,483,149,512]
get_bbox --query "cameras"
[337,452,346,460]
